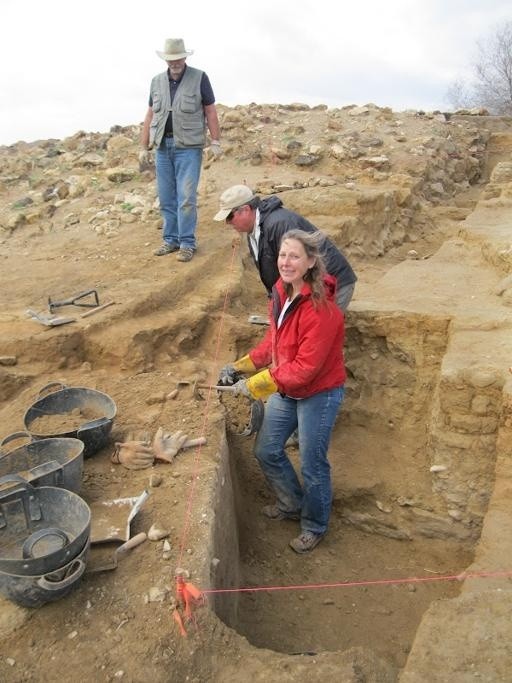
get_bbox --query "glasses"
[226,207,239,223]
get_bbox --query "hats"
[212,184,255,222]
[155,38,194,62]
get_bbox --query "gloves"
[229,367,279,407]
[203,138,224,161]
[216,353,259,388]
[137,145,153,172]
[110,428,188,471]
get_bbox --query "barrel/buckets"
[0,379,116,609]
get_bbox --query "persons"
[140,39,221,261]
[212,185,357,447]
[220,230,348,554]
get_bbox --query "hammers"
[88,534,146,577]
[190,382,238,400]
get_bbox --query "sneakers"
[150,243,179,256]
[288,528,326,553]
[258,503,302,523]
[175,244,195,262]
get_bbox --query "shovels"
[87,489,152,544]
